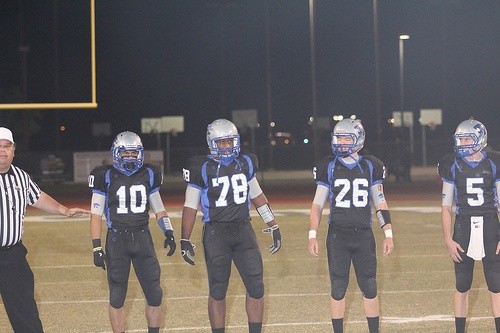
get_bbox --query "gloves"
[92,238,106,272]
[180,238,195,266]
[164,230,176,256]
[261,224,281,254]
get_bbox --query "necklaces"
[0,180,15,195]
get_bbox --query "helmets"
[330,118,365,157]
[109,131,144,176]
[453,119,487,158]
[206,119,241,165]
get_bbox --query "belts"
[0,240,21,250]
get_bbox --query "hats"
[0,126,14,145]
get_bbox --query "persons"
[181,119,282,333]
[0,127,92,333]
[88,131,176,333]
[436,119,500,333]
[307,119,394,333]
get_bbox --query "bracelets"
[385,229,393,238]
[308,230,316,238]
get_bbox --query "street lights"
[399,35,411,145]
